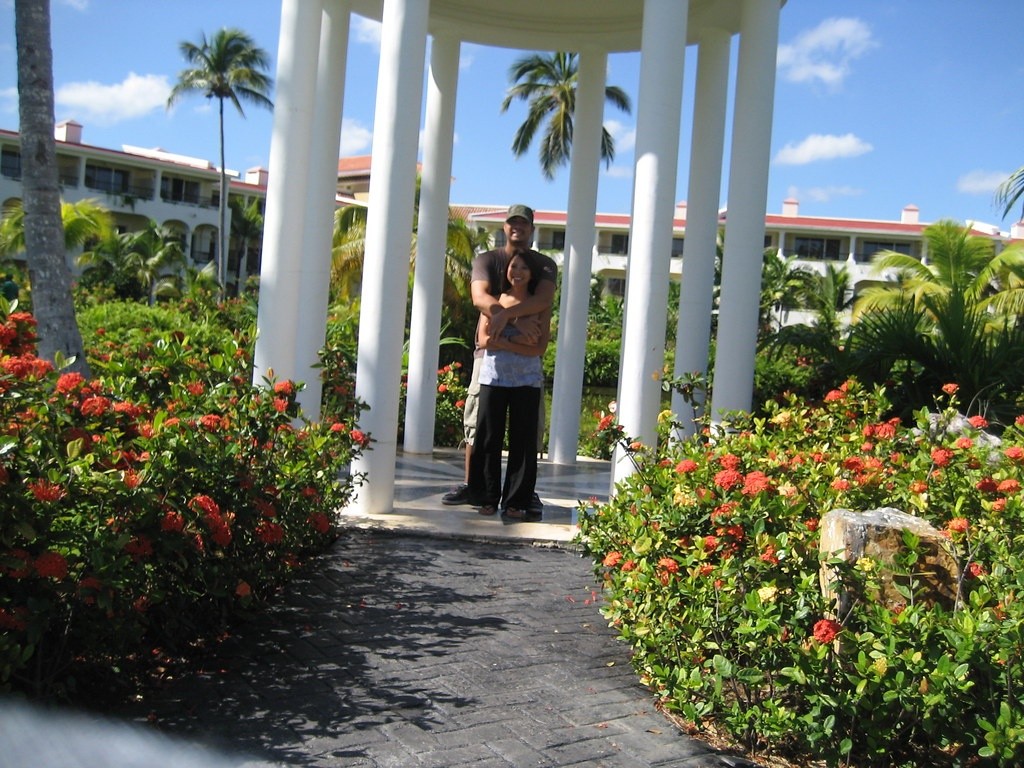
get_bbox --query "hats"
[506,204,533,224]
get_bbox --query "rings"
[486,323,489,325]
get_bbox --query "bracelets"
[511,317,518,326]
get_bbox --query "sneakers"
[528,492,543,508]
[441,484,468,505]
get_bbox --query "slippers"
[505,507,524,518]
[480,503,494,515]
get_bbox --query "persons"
[441,206,557,520]
